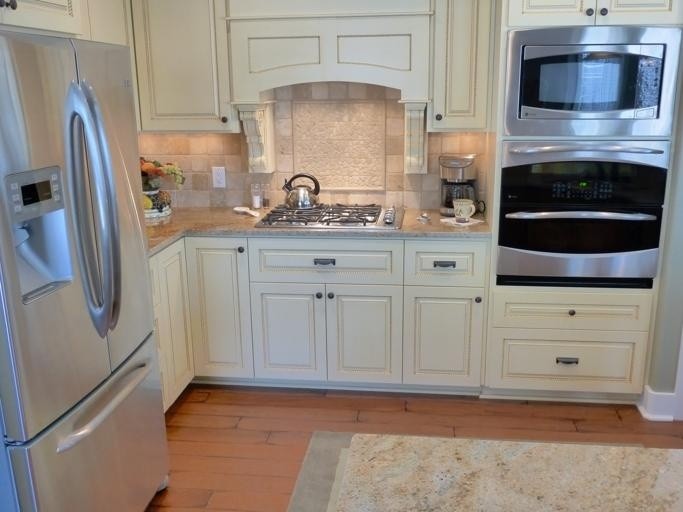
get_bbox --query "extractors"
[226,2,431,176]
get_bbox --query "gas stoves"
[252,203,406,232]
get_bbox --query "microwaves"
[518,42,667,123]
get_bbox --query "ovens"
[492,137,675,289]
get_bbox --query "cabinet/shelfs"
[397,230,495,403]
[87,2,133,45]
[480,279,660,407]
[182,230,253,390]
[424,1,502,136]
[149,235,197,414]
[0,0,95,39]
[247,230,397,398]
[125,1,245,140]
[495,1,683,31]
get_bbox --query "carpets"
[280,425,683,512]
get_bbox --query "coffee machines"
[437,151,479,216]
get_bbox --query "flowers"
[140,151,186,191]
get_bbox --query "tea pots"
[280,173,320,207]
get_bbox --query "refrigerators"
[0,23,174,511]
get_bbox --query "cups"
[249,183,261,210]
[259,182,271,209]
[451,198,475,223]
